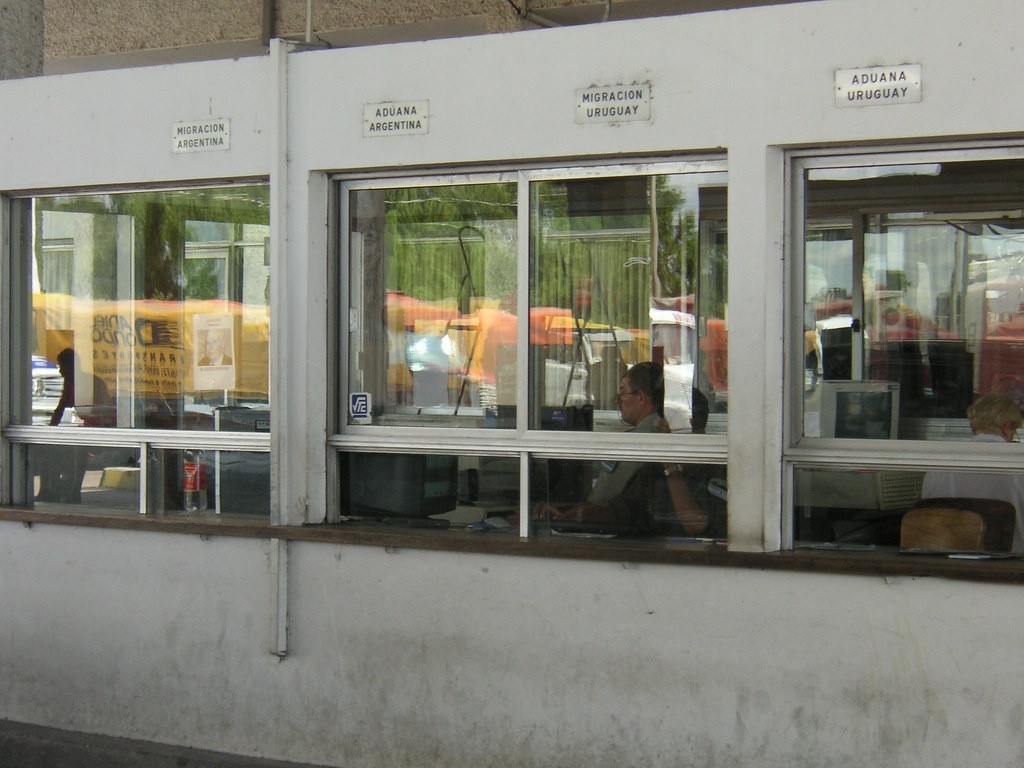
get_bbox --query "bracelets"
[664,463,683,475]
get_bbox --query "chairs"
[900,497,1017,554]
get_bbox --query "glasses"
[1011,431,1021,444]
[616,391,640,401]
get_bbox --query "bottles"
[183,450,207,512]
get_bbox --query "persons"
[49,348,110,426]
[200,330,232,364]
[533,270,1024,557]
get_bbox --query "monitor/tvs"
[355,452,457,515]
[478,403,596,506]
[801,381,900,439]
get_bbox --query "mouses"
[484,516,509,530]
